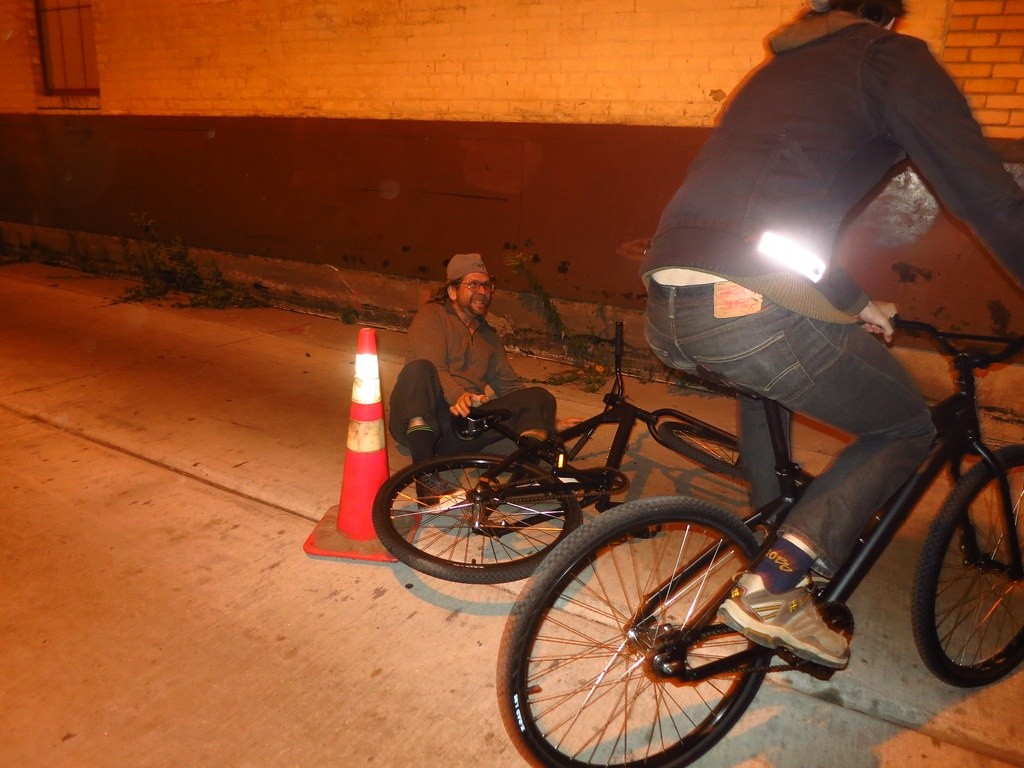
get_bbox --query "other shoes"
[416,472,467,514]
[509,466,556,483]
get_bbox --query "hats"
[447,253,489,284]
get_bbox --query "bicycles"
[495,318,1024,768]
[371,319,815,585]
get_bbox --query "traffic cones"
[304,330,424,565]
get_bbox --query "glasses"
[455,280,495,293]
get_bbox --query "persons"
[638,0,1024,670]
[388,253,557,512]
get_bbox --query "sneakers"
[716,569,851,669]
